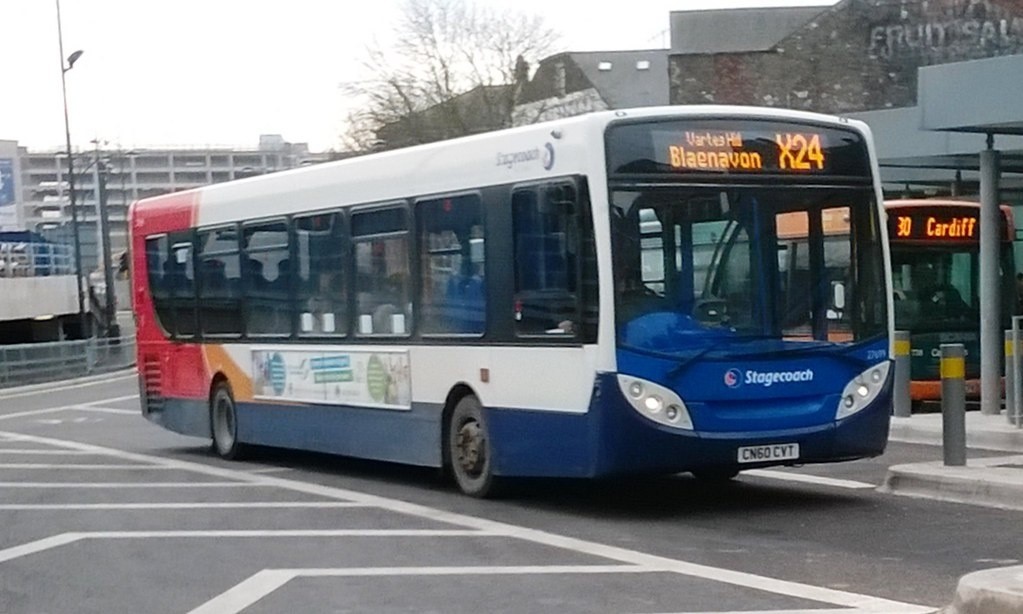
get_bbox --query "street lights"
[57,0,88,340]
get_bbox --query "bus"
[130,103,901,503]
[459,199,1016,406]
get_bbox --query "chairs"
[456,279,486,330]
[516,235,578,290]
[163,259,401,333]
